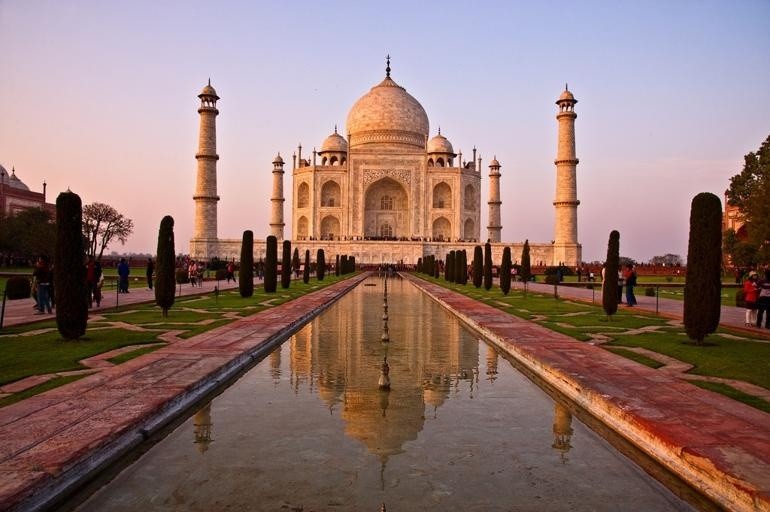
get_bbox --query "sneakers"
[745,323,755,328]
[191,281,237,289]
[119,288,154,294]
[756,323,770,329]
[31,296,105,315]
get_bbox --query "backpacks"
[233,275,236,283]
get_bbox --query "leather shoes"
[624,303,637,307]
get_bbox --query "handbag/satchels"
[96,273,105,289]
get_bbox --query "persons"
[743,270,759,327]
[29,253,103,315]
[626,263,637,307]
[755,267,770,330]
[617,265,624,304]
[195,262,204,288]
[145,258,154,291]
[188,260,198,287]
[601,262,607,304]
[225,262,236,284]
[116,258,131,293]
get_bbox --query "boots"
[259,275,262,280]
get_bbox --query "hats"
[747,271,758,279]
